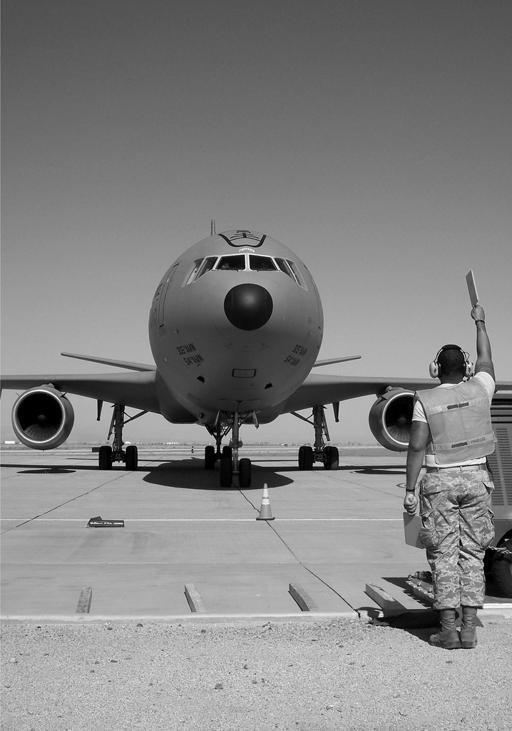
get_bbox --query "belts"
[426,463,487,475]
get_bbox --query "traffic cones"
[255,484,274,520]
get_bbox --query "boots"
[428,606,477,650]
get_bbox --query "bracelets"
[474,318,485,326]
[405,487,415,494]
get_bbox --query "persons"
[402,305,500,651]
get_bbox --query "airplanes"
[1,219,512,487]
